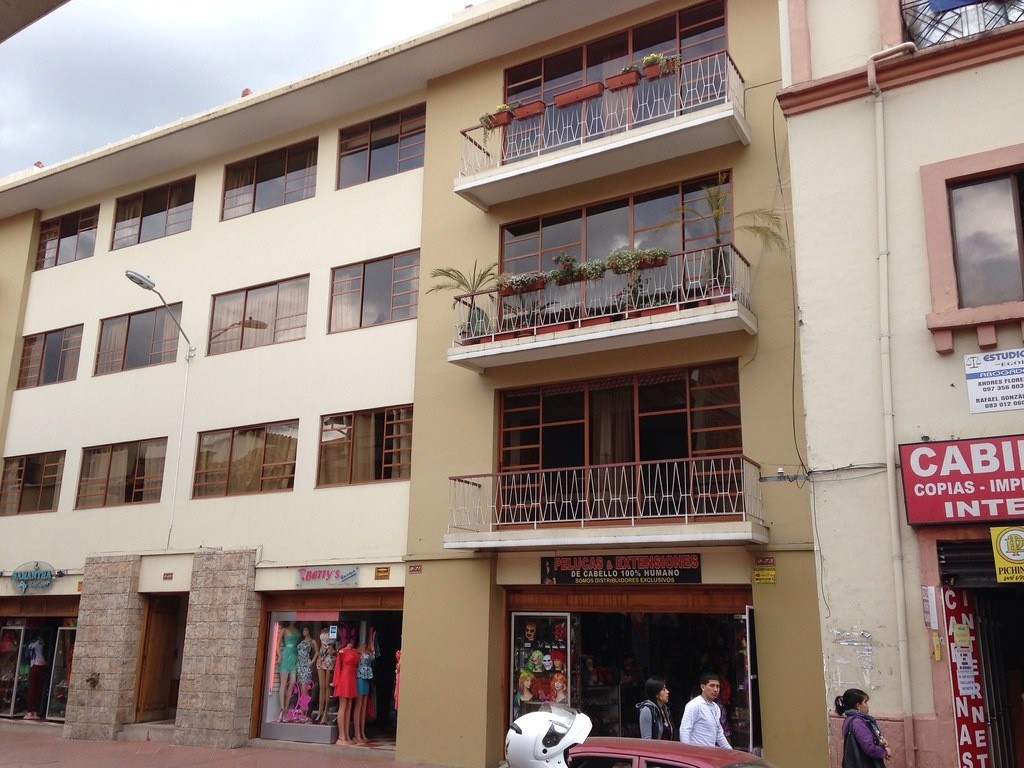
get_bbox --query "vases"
[535,323,572,335]
[642,58,674,82]
[558,268,586,286]
[484,111,514,130]
[552,81,604,110]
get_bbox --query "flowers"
[478,102,511,146]
[546,247,604,285]
[642,53,682,79]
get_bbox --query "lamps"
[211,317,269,344]
[124,269,196,360]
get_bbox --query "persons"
[23,636,48,720]
[393,635,403,751]
[635,676,677,741]
[679,673,733,749]
[834,687,891,768]
[275,621,376,745]
[518,621,632,704]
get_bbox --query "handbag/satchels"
[841,716,887,768]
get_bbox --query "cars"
[568,736,778,768]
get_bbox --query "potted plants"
[497,271,549,298]
[421,256,499,346]
[604,63,642,92]
[611,289,640,320]
[653,171,787,305]
[607,247,671,278]
[693,283,710,307]
[574,314,613,330]
[481,327,516,344]
[486,291,559,338]
[512,99,544,120]
[639,295,684,316]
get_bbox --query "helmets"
[504,700,593,768]
